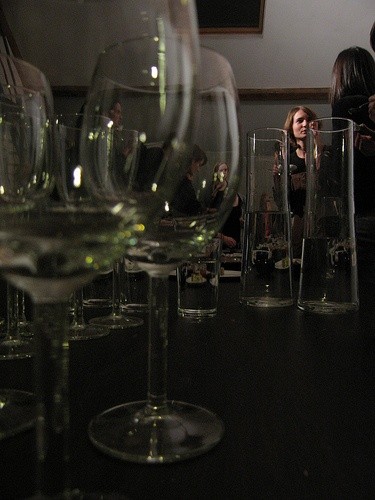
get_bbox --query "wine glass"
[0,0,244,500]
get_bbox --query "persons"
[138,133,186,191]
[314,45,375,239]
[169,144,217,220]
[253,193,277,276]
[273,106,328,258]
[353,20,375,160]
[75,97,133,190]
[200,160,243,255]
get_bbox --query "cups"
[297,116,359,315]
[238,128,296,309]
